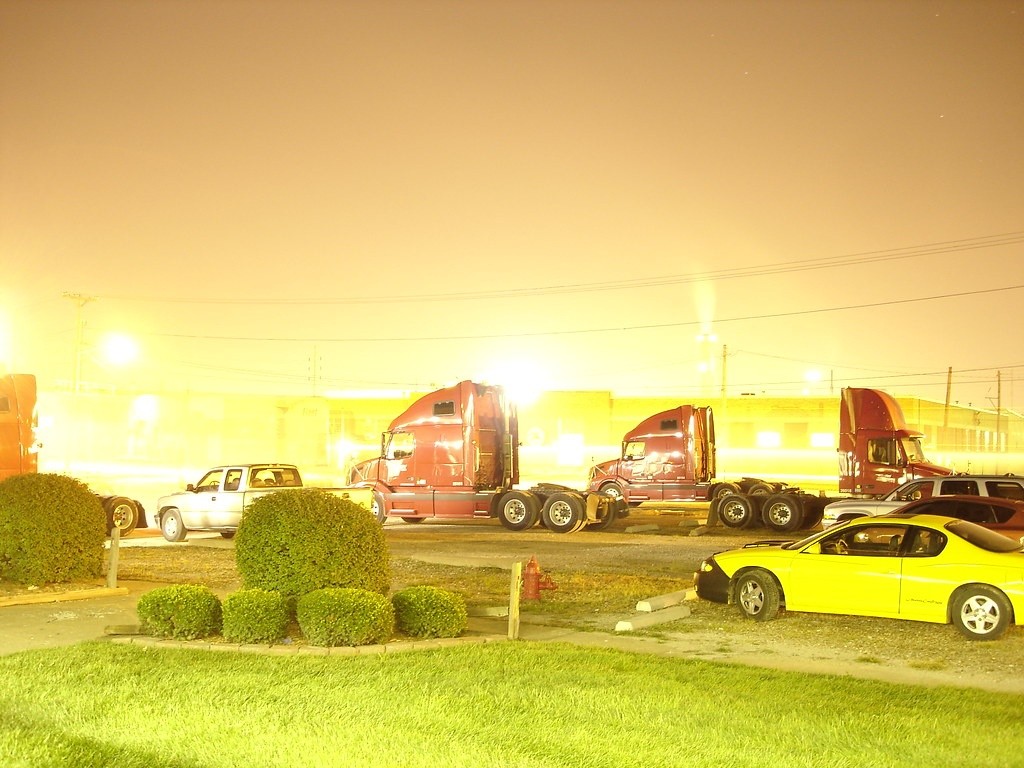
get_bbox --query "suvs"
[821,476,1024,531]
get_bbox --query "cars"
[854,495,1024,546]
[693,514,1024,641]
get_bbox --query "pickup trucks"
[154,463,372,542]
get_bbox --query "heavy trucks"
[347,381,630,534]
[706,384,960,533]
[0,373,148,538]
[587,404,800,508]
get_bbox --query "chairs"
[911,533,940,554]
[887,534,902,551]
[229,478,240,490]
